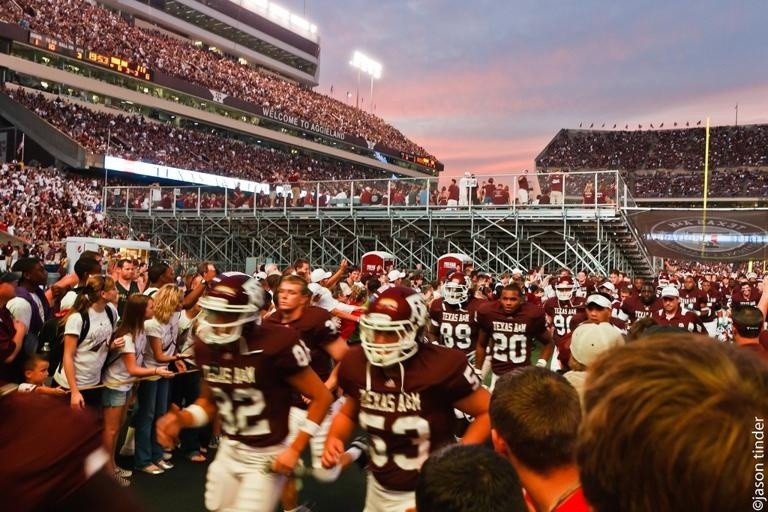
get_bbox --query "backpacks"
[34,306,114,377]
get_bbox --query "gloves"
[535,358,547,369]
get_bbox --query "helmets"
[195,270,266,344]
[442,272,470,305]
[555,276,574,302]
[356,287,429,369]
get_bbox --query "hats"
[598,282,614,292]
[660,287,680,299]
[584,294,612,311]
[309,268,332,283]
[569,322,623,368]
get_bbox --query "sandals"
[185,448,208,464]
[135,460,175,478]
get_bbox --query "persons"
[0,1,768,511]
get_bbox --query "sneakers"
[109,466,132,487]
[121,445,137,457]
